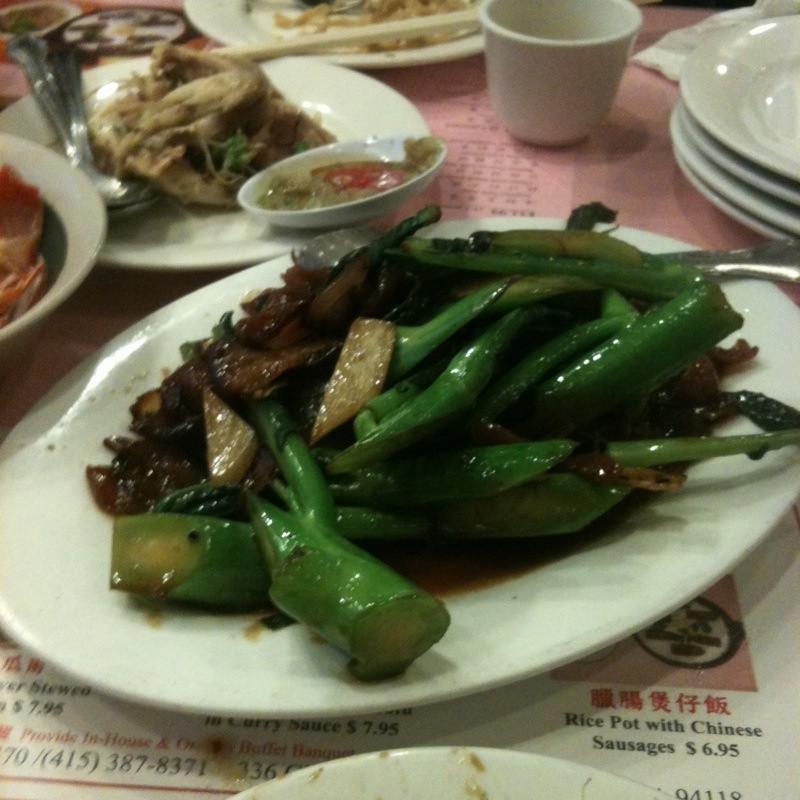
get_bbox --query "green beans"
[110,202,800,678]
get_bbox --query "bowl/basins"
[0,131,111,348]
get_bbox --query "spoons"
[6,32,161,223]
[289,225,800,283]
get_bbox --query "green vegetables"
[209,129,441,192]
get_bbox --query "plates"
[0,212,800,719]
[181,0,484,69]
[225,744,677,800]
[236,134,447,228]
[0,51,430,270]
[667,13,800,246]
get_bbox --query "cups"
[479,0,643,148]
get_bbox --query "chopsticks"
[207,8,479,58]
